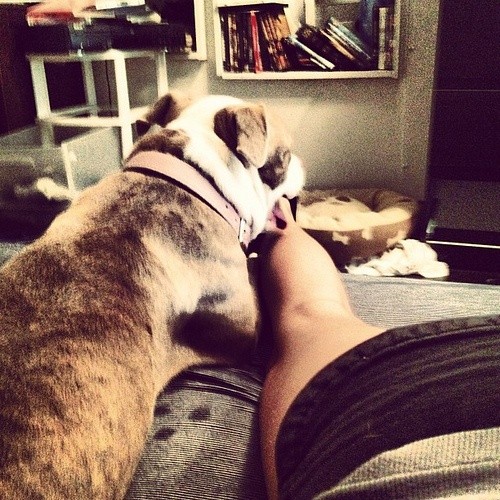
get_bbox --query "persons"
[247,197,500,500]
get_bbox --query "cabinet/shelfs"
[214,1,400,80]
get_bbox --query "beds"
[0,241,499,500]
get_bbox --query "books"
[218,3,392,73]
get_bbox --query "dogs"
[0,92,307,500]
